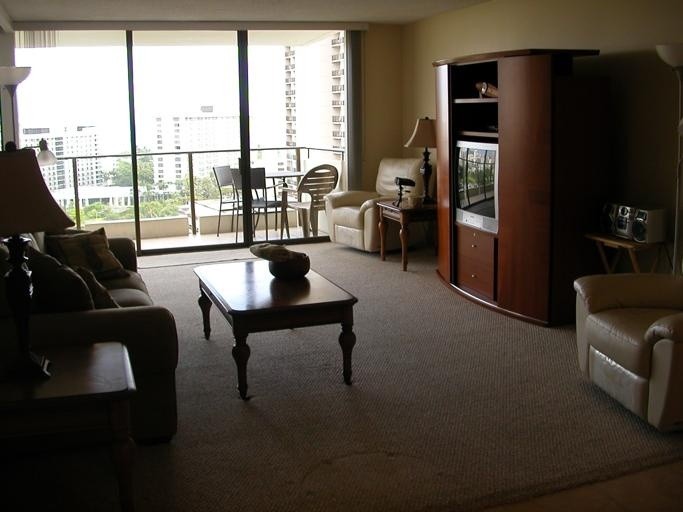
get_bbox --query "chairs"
[214,165,258,237]
[232,167,291,241]
[280,163,338,238]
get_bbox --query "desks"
[260,170,308,237]
[585,226,668,274]
[0,341,141,510]
[374,196,439,270]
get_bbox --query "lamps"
[401,114,437,204]
[21,137,57,167]
[0,150,77,379]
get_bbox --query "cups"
[480,81,498,98]
[407,195,421,208]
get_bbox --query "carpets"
[127,243,680,509]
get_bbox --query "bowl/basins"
[268,252,310,281]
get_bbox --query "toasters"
[600,200,668,245]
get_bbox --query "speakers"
[614,204,640,240]
[632,206,666,243]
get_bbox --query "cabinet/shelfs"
[429,48,616,328]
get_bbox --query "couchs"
[322,154,429,255]
[568,272,681,435]
[23,225,179,442]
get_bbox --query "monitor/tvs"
[454,139,500,236]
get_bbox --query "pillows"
[73,263,121,308]
[48,227,130,278]
[23,243,96,309]
